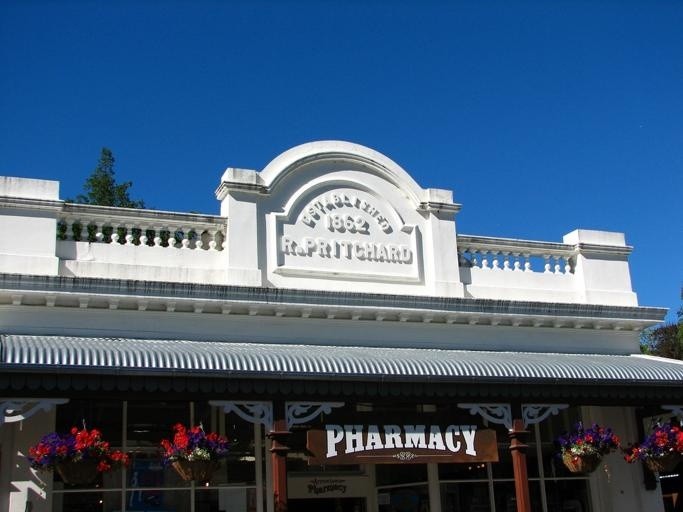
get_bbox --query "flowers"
[27,427,130,471]
[558,424,621,483]
[160,424,232,461]
[621,417,683,464]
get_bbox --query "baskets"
[563,454,602,472]
[646,455,681,472]
[60,462,95,484]
[173,460,221,481]
